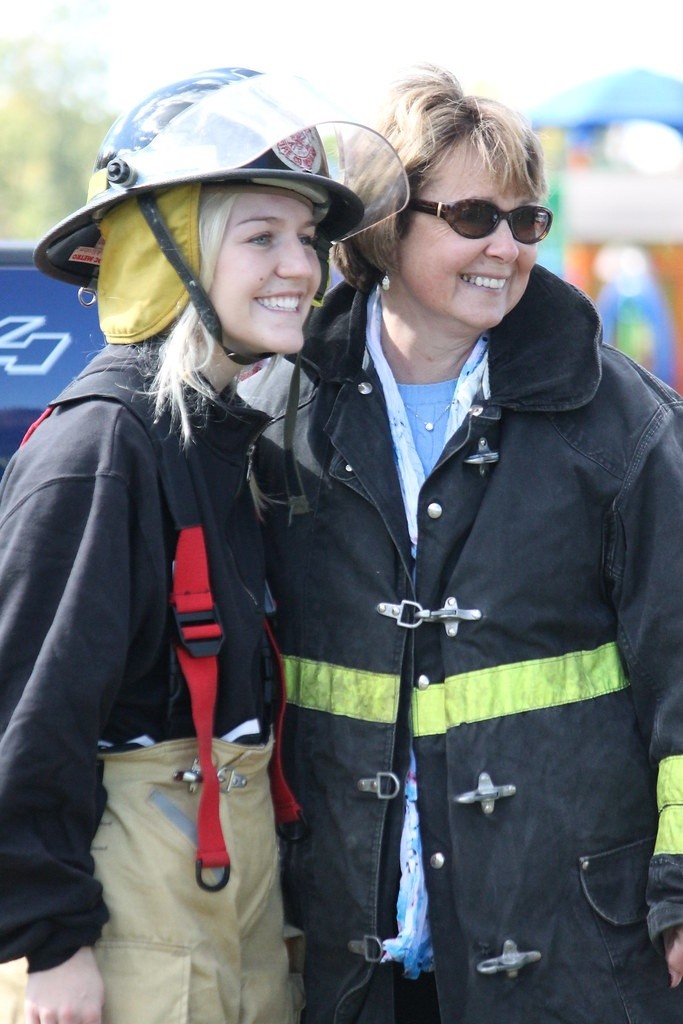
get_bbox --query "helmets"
[33,66,410,286]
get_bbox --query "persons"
[238,69,683,1024]
[0,70,414,1024]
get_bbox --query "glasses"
[408,198,553,244]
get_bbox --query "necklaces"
[403,402,452,431]
